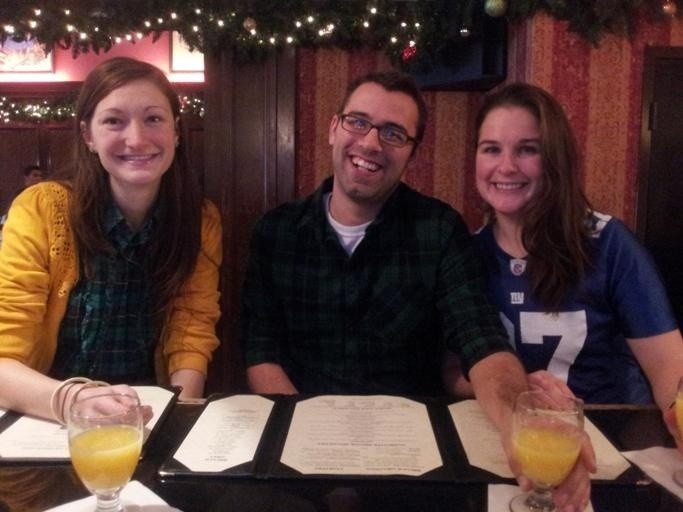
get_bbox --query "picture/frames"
[0,35,56,73]
[170,31,205,75]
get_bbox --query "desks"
[0,404,683,512]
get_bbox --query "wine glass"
[66,388,144,511]
[672,376,683,488]
[509,392,583,511]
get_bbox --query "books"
[154,382,654,492]
[0,385,182,466]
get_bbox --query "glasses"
[335,111,421,148]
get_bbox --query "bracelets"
[65,379,111,427]
[49,376,93,427]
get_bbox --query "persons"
[240,70,602,511]
[432,80,681,456]
[0,57,227,432]
[11,164,41,203]
